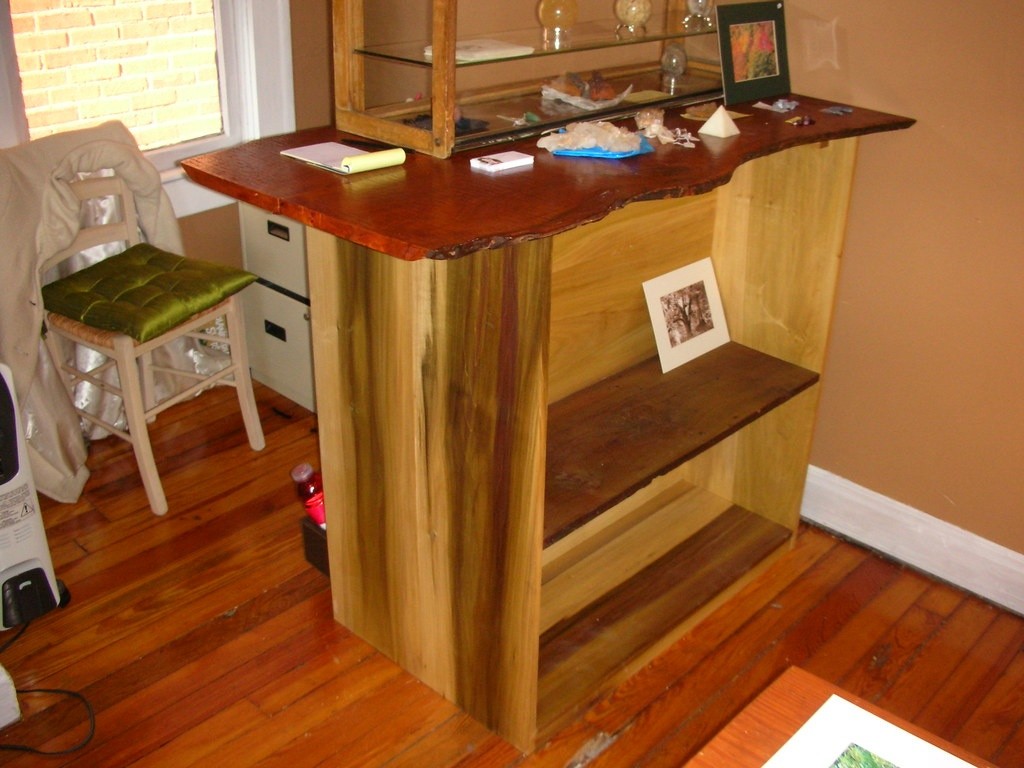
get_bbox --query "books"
[424,38,535,61]
[280,142,407,174]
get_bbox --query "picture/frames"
[715,0,791,106]
[641,257,731,374]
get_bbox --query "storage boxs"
[300,517,329,577]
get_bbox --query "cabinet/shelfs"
[180,65,916,759]
[332,0,725,160]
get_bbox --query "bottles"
[289,461,327,532]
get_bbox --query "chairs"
[1,122,266,517]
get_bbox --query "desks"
[683,666,1004,768]
